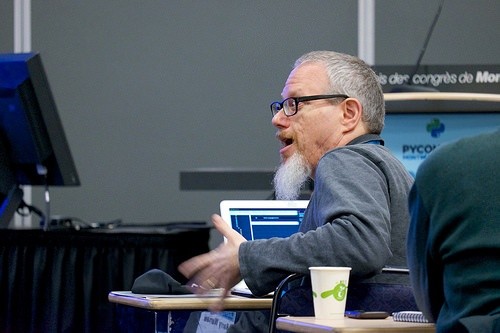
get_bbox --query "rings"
[206,278,216,288]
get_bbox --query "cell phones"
[346,310,389,319]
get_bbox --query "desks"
[109,291,274,310]
[275,316,438,333]
[0,224,211,333]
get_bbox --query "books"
[393,311,430,323]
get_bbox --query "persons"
[179,49,415,333]
[407,128,500,333]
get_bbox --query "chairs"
[269,267,420,333]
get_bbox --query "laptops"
[219,200,311,297]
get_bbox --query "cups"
[309,266,351,319]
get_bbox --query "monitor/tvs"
[0,52,81,228]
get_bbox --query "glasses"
[270,94,349,117]
